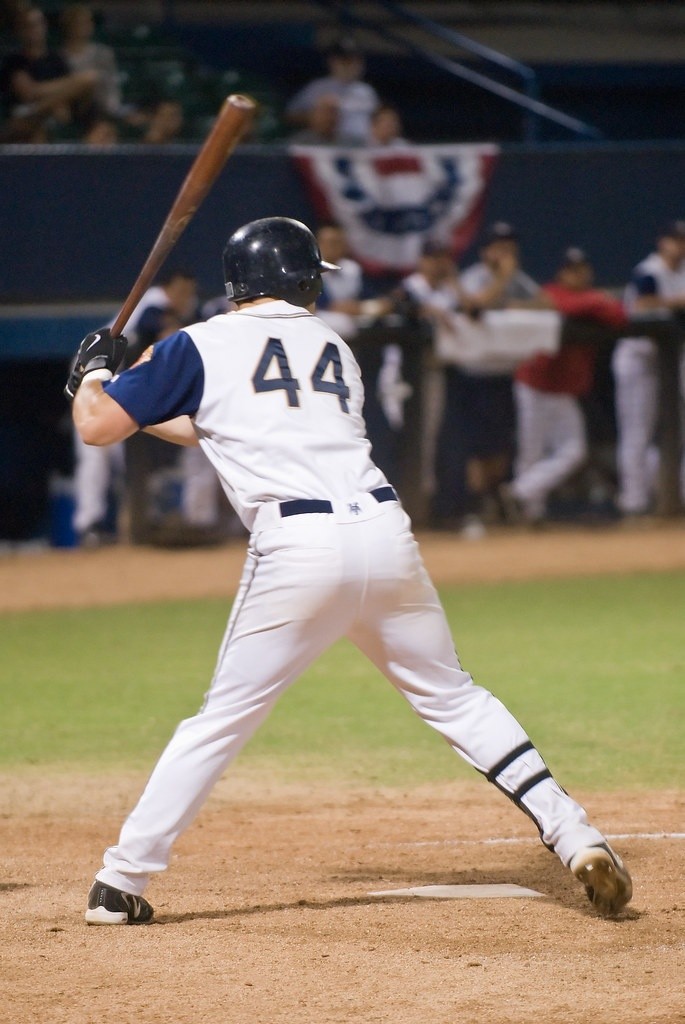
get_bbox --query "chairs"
[97,19,291,144]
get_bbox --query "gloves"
[64,357,82,402]
[78,328,128,383]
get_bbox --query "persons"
[179,295,228,547]
[313,226,363,312]
[62,216,635,925]
[0,0,182,146]
[364,220,685,529]
[71,271,199,550]
[286,37,411,149]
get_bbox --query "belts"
[279,487,398,517]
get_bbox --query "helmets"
[224,216,342,306]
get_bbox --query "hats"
[478,222,516,242]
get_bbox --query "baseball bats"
[80,93,255,371]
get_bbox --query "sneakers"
[568,843,633,914]
[86,880,154,925]
